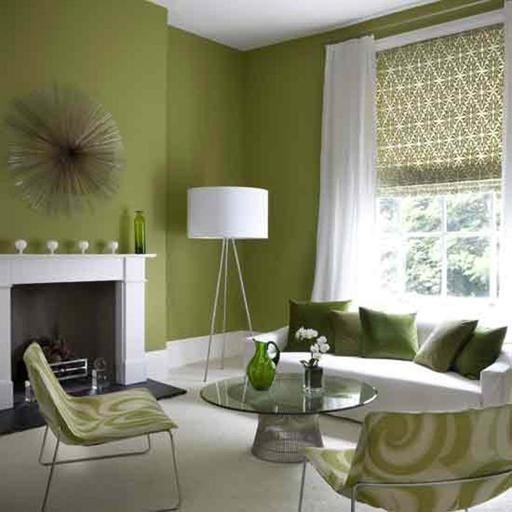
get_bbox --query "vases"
[304,366,324,388]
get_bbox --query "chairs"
[300,403,512,511]
[24,342,182,512]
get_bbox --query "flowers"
[294,326,330,367]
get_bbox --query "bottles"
[134,210,146,254]
[246,339,281,391]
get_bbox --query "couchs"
[247,317,512,424]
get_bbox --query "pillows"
[282,298,352,353]
[451,325,508,380]
[359,307,419,360]
[413,319,479,372]
[330,309,360,357]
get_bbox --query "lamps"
[187,186,269,385]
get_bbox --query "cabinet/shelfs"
[0,253,158,410]
